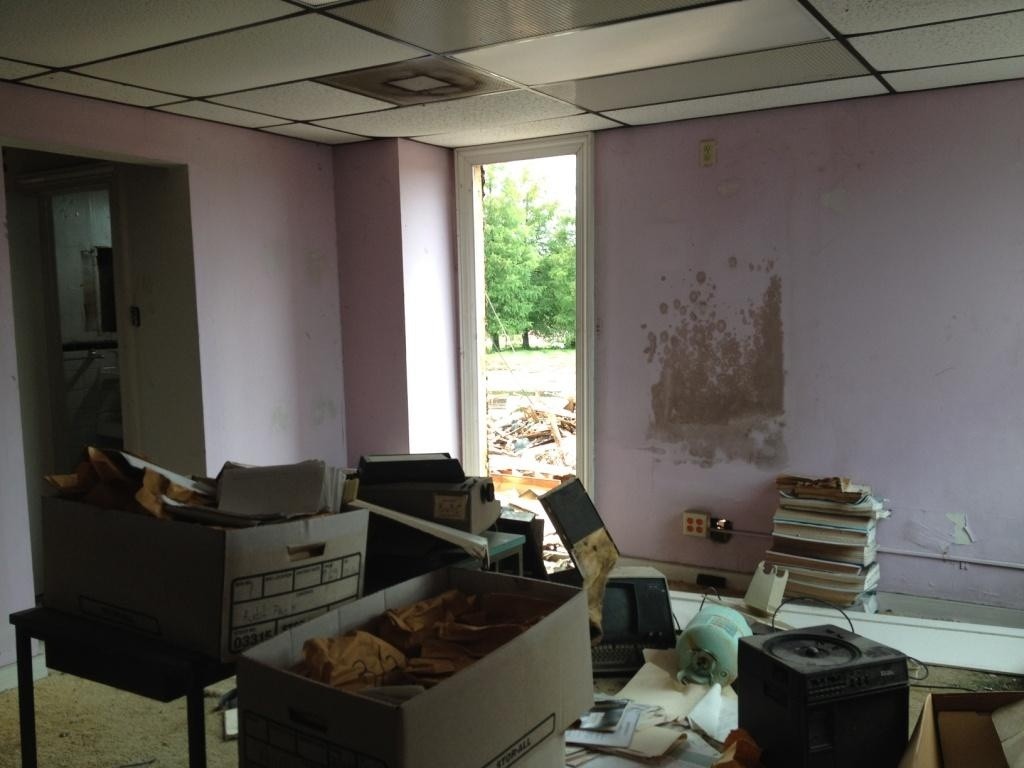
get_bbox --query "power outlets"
[683,511,711,538]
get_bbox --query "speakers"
[740,623,909,768]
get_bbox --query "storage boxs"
[895,688,1024,768]
[38,446,600,768]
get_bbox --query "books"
[766,475,893,613]
[164,458,346,528]
[343,479,488,559]
